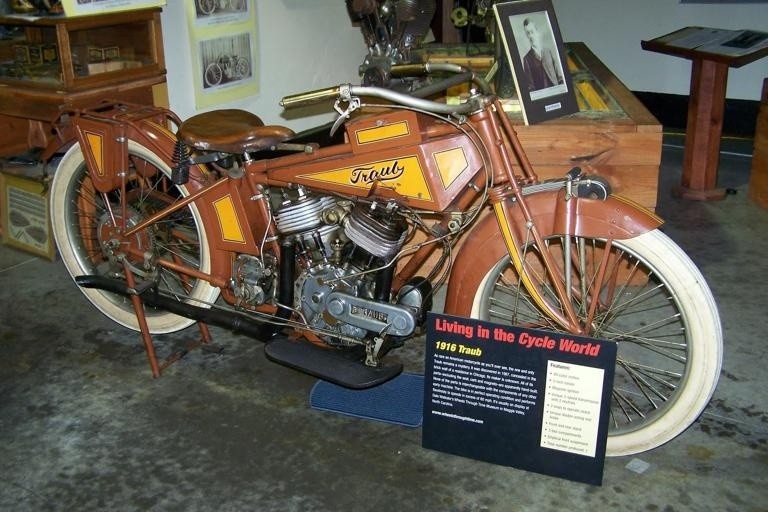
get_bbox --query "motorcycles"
[37,1,725,470]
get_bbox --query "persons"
[518,15,565,92]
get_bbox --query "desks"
[641,26,768,202]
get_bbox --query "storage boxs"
[377,39,664,288]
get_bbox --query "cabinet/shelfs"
[0,5,169,182]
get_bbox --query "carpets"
[309,360,433,430]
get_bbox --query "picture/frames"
[490,0,579,128]
[0,170,56,264]
